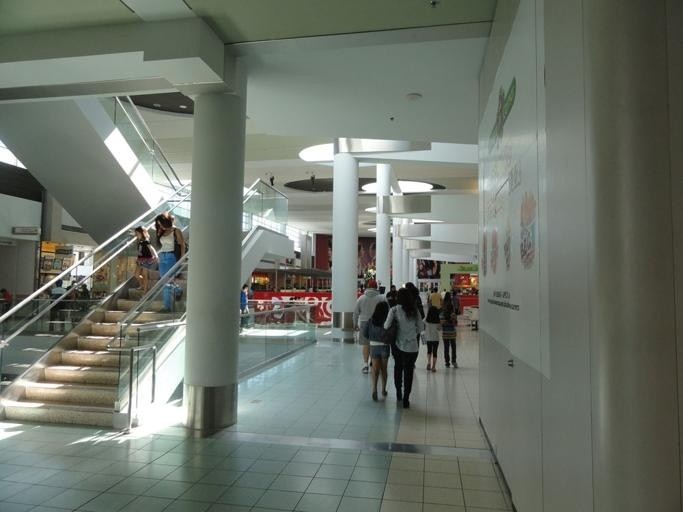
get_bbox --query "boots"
[394,365,414,409]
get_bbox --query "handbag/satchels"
[173,228,189,262]
[382,306,397,344]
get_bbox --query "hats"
[368,279,377,288]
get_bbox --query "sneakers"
[156,289,183,313]
[362,366,369,374]
[427,362,459,372]
[372,391,387,400]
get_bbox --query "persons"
[156,211,185,314]
[352,279,463,408]
[135,226,158,294]
[240,284,249,327]
[49,280,90,332]
[0,288,12,312]
